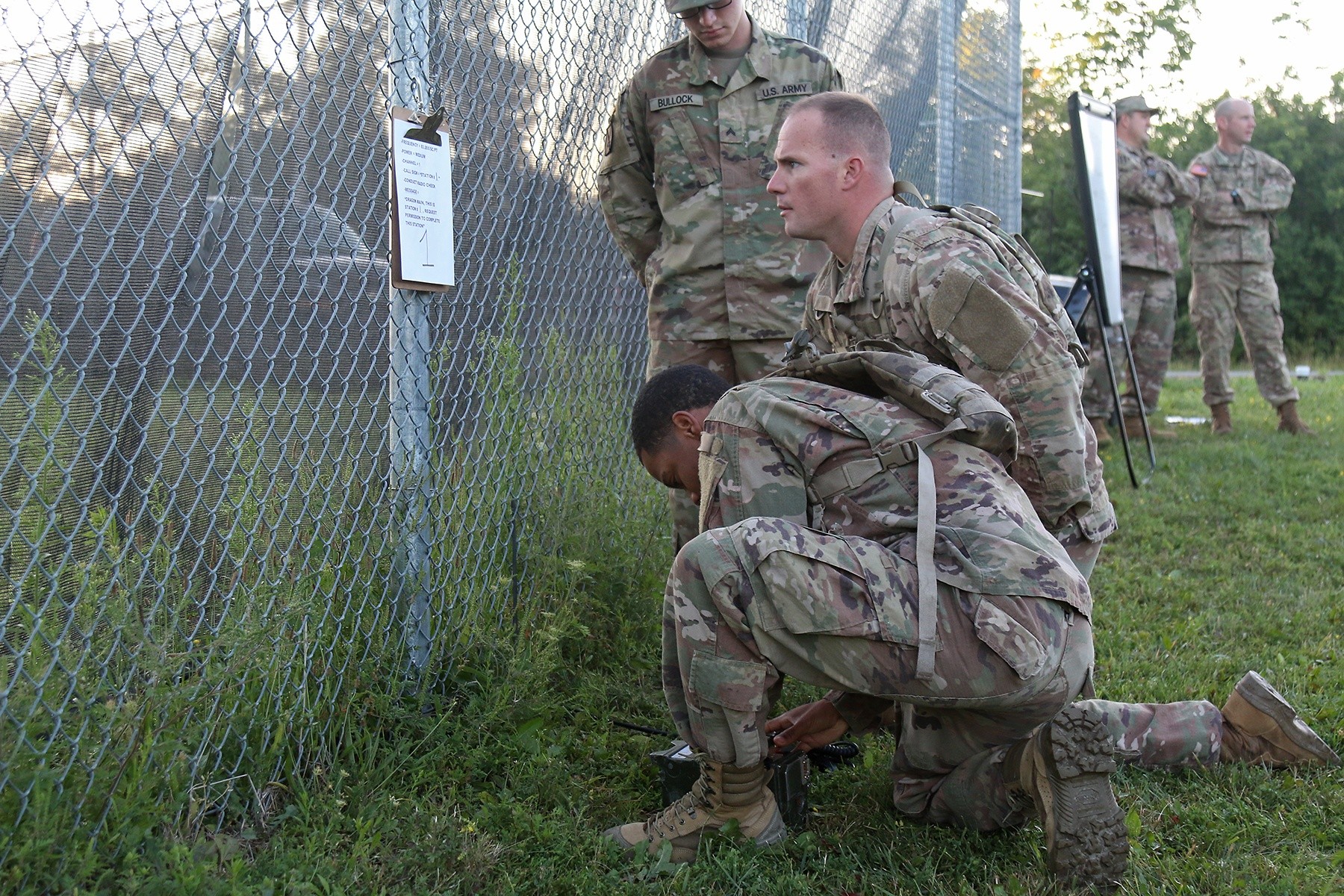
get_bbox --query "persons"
[766,93,1344,778]
[594,0,847,560]
[598,366,1130,896]
[1079,94,1201,446]
[1181,96,1319,439]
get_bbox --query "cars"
[174,194,390,338]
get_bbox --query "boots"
[1210,403,1234,436]
[1220,670,1343,770]
[1116,415,1178,440]
[1088,416,1113,447]
[603,747,788,868]
[1003,708,1130,896]
[1278,400,1320,438]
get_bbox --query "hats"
[664,0,721,14]
[1113,96,1158,118]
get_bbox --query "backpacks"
[712,334,1019,501]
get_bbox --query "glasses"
[674,0,732,19]
